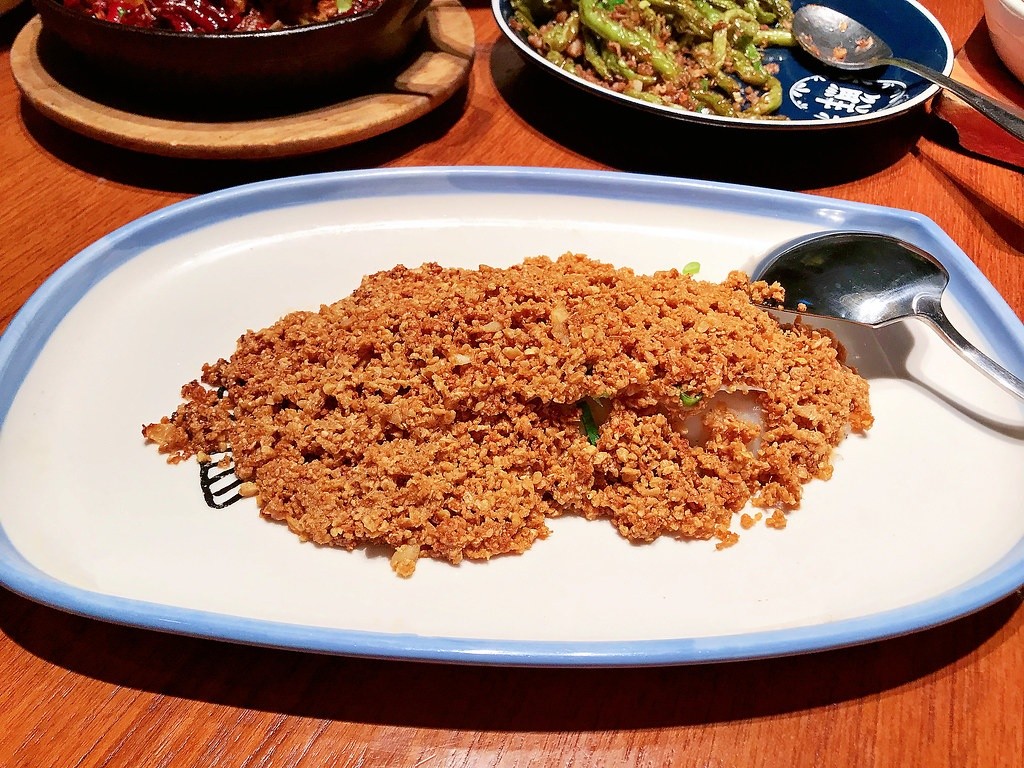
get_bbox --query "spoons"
[792,4,1024,143]
[746,231,1023,402]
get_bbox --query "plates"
[491,0,953,130]
[2,164,1022,668]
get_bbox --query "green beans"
[509,0,797,118]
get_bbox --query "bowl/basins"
[30,0,431,100]
[984,1,1023,83]
